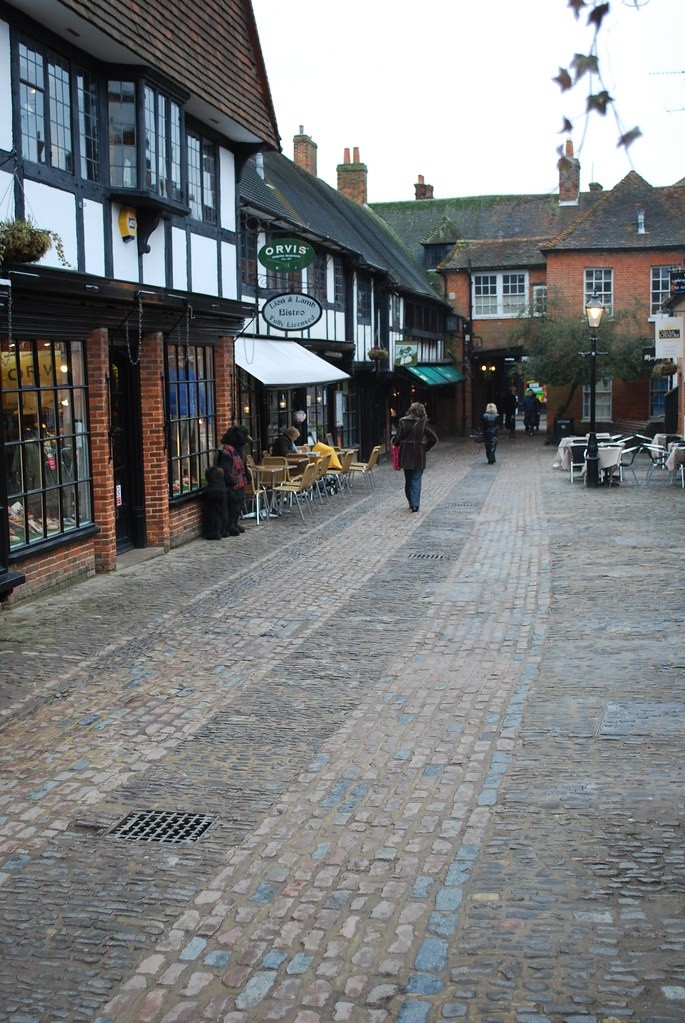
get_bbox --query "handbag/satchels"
[392,445,402,471]
[475,433,485,443]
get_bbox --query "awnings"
[233,336,352,392]
[402,365,468,388]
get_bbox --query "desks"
[286,456,310,464]
[258,465,297,514]
[652,433,680,466]
[554,435,614,467]
[248,463,283,519]
[668,446,685,487]
[559,442,625,482]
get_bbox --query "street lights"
[578,288,609,486]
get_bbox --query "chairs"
[555,431,685,492]
[241,445,382,525]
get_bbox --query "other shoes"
[488,459,496,464]
[409,504,419,512]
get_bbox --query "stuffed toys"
[204,424,253,541]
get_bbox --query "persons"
[478,403,502,464]
[392,402,439,513]
[271,428,300,457]
[501,387,542,438]
